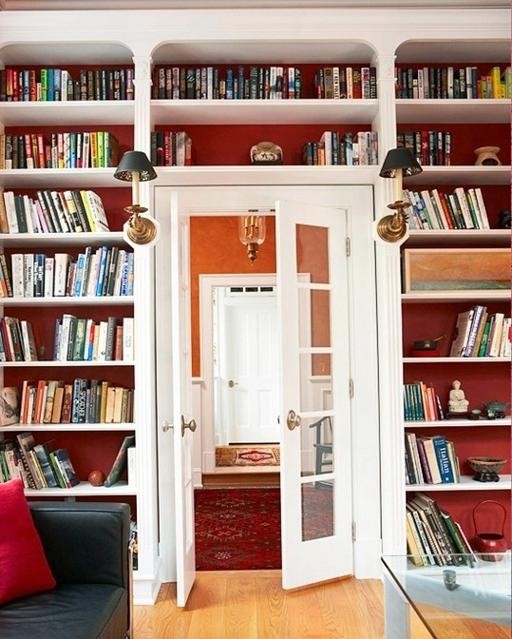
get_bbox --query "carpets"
[193,488,332,572]
[215,447,280,467]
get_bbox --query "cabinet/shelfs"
[0,6,512,605]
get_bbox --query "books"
[404,432,460,485]
[2,377,133,425]
[150,64,379,165]
[0,316,37,361]
[103,436,135,487]
[404,381,444,421]
[2,132,119,170]
[1,433,80,488]
[2,246,134,297]
[450,306,511,357]
[394,65,511,99]
[1,190,109,233]
[402,187,490,230]
[406,492,478,566]
[397,131,451,165]
[54,314,134,360]
[131,521,138,572]
[1,68,135,101]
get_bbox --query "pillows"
[0,478,57,605]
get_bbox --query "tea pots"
[480,400,510,418]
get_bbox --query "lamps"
[377,147,423,244]
[113,150,157,245]
[237,212,266,262]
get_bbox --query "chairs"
[308,416,335,489]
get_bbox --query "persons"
[448,381,469,412]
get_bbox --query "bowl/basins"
[468,456,507,474]
[413,340,437,351]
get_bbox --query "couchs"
[0,500,135,639]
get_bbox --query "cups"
[472,409,482,414]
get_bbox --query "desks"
[379,552,511,639]
